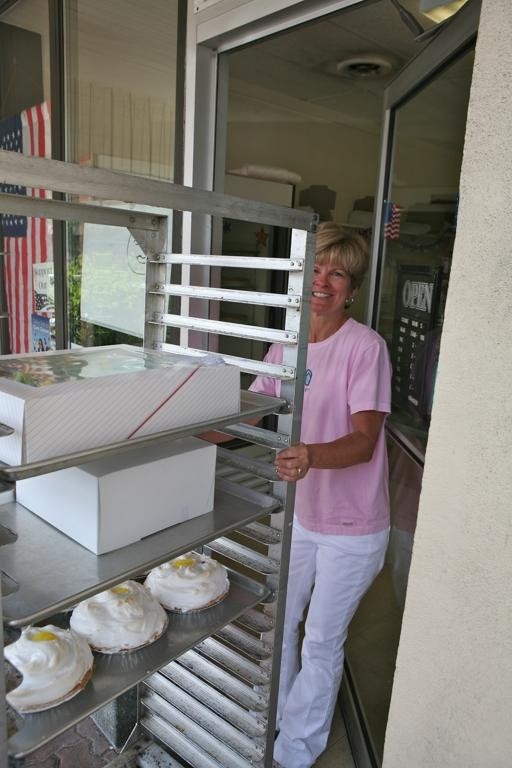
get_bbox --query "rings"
[295,466,304,478]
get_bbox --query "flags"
[377,199,403,243]
[2,99,57,356]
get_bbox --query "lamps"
[337,43,392,79]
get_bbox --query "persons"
[182,218,397,768]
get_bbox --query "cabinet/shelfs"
[0,149,319,768]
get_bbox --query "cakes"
[143,550,230,614]
[70,580,169,655]
[3,623,95,715]
